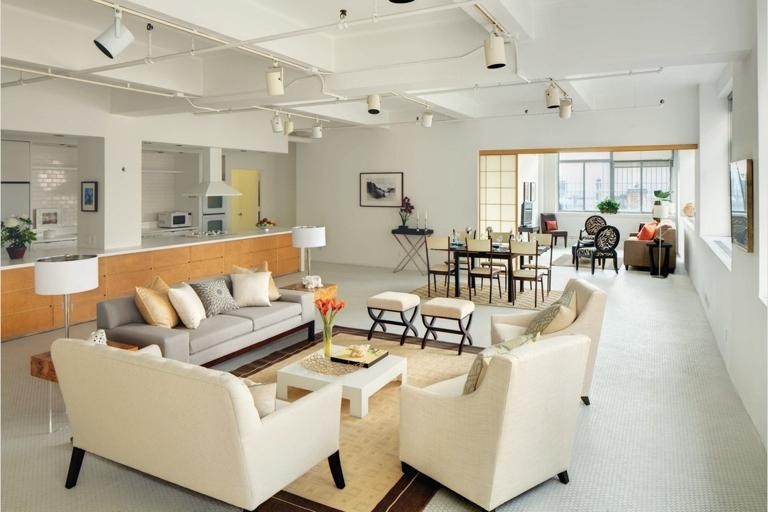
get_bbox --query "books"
[331,345,389,368]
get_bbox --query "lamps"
[545,83,561,109]
[271,112,284,133]
[93,8,135,59]
[265,61,284,97]
[421,106,433,128]
[311,119,323,139]
[483,24,507,70]
[653,205,668,278]
[367,95,382,114]
[559,93,572,119]
[34,253,98,337]
[284,114,294,136]
[292,225,326,275]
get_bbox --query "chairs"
[575,225,620,274]
[465,237,501,303]
[425,235,455,298]
[509,239,544,307]
[540,212,568,248]
[444,228,476,286]
[480,230,514,292]
[521,232,553,296]
[579,215,607,240]
[572,239,594,264]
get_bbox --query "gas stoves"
[180,230,235,240]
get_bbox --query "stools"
[646,241,672,275]
[31,337,138,433]
[280,282,337,300]
[421,297,476,355]
[367,290,421,346]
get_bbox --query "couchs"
[97,272,314,366]
[50,337,346,511]
[623,222,677,274]
[398,335,592,512]
[491,277,608,406]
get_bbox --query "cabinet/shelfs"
[0,228,300,343]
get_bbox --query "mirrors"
[730,159,754,253]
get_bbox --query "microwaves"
[158,210,193,228]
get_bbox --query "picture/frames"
[524,182,530,201]
[81,181,98,212]
[530,182,536,201]
[360,172,404,207]
[36,208,63,229]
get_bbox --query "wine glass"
[496,236,503,251]
[516,235,522,242]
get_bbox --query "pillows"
[545,221,557,230]
[461,331,540,395]
[167,281,206,328]
[247,380,277,418]
[190,279,239,318]
[639,224,656,240]
[134,275,180,328]
[232,261,281,301]
[637,220,657,237]
[524,289,577,335]
[230,271,273,307]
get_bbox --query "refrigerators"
[229,168,259,232]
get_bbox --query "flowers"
[1,215,37,247]
[400,196,415,214]
[313,297,345,325]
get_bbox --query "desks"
[518,226,540,242]
[391,229,434,276]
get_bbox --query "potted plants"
[597,199,620,214]
[654,190,670,244]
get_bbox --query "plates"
[492,244,502,248]
[257,228,274,233]
[451,242,465,246]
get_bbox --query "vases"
[398,213,411,228]
[5,247,27,259]
[323,325,332,356]
